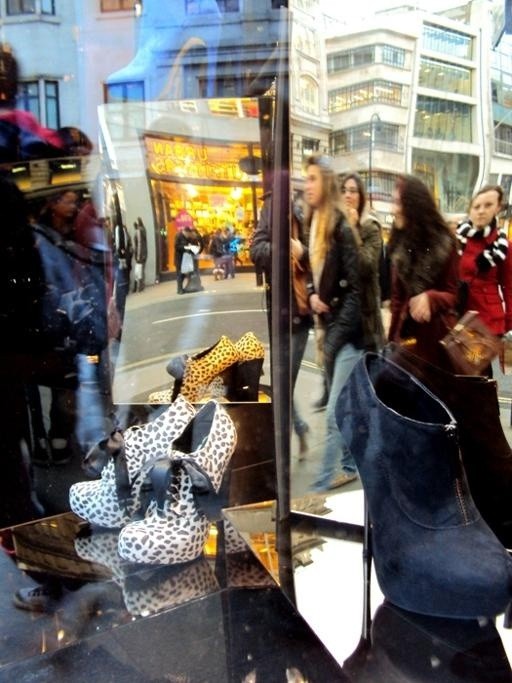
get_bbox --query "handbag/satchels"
[439,308,502,374]
[134,264,143,282]
[180,253,194,274]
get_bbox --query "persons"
[310,173,387,412]
[1,171,146,529]
[250,171,313,463]
[2,522,84,610]
[294,155,385,493]
[453,185,511,415]
[380,173,460,373]
[174,224,241,295]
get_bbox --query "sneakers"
[11,586,58,612]
[331,470,357,488]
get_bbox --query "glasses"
[340,185,357,193]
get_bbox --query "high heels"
[342,554,511,683]
[117,400,237,565]
[73,528,119,574]
[209,332,264,401]
[148,335,239,402]
[118,531,227,616]
[107,2,220,98]
[335,352,512,619]
[381,343,511,549]
[226,514,275,587]
[69,394,196,529]
[298,424,311,460]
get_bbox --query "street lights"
[238,143,265,288]
[368,113,383,213]
